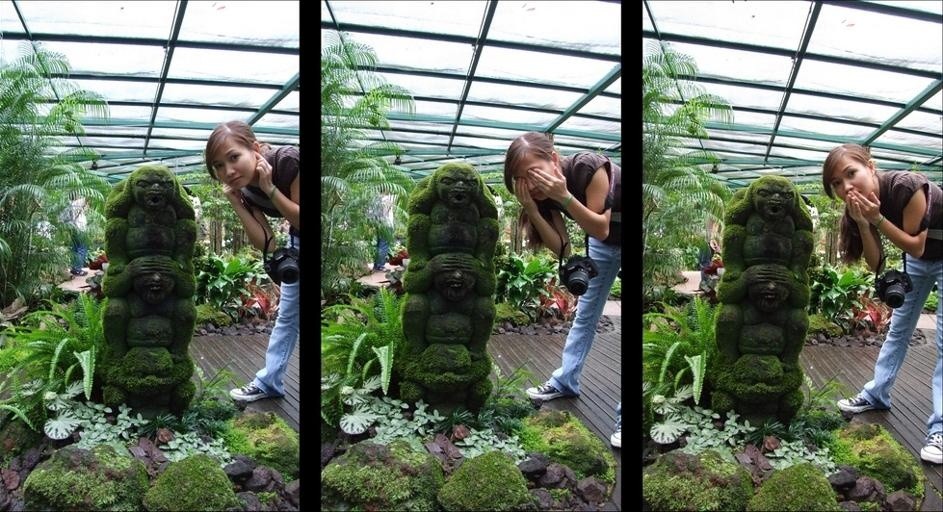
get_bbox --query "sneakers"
[228,380,267,403]
[610,414,624,449]
[524,381,564,403]
[919,431,941,464]
[835,395,876,415]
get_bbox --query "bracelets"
[563,193,573,212]
[269,185,276,201]
[876,216,886,229]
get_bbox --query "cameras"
[875,269,913,308]
[264,247,300,284]
[559,257,598,295]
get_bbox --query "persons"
[502,131,620,403]
[205,120,299,403]
[697,218,722,292]
[58,193,89,277]
[822,144,942,466]
[367,187,398,271]
